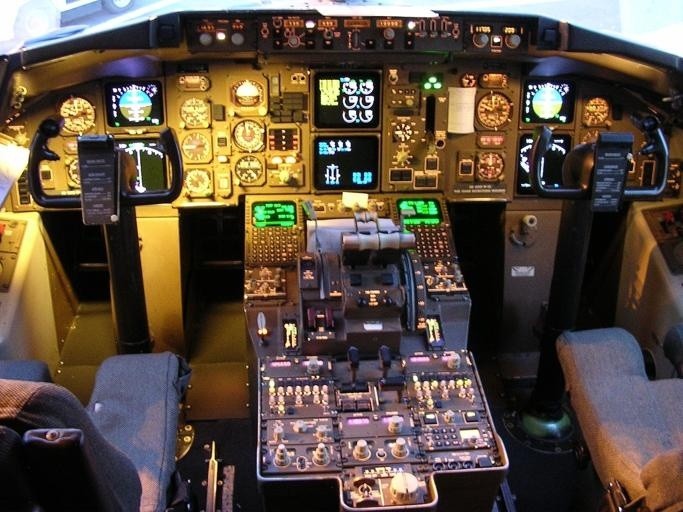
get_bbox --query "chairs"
[555,327,683,512]
[0,352,191,512]
